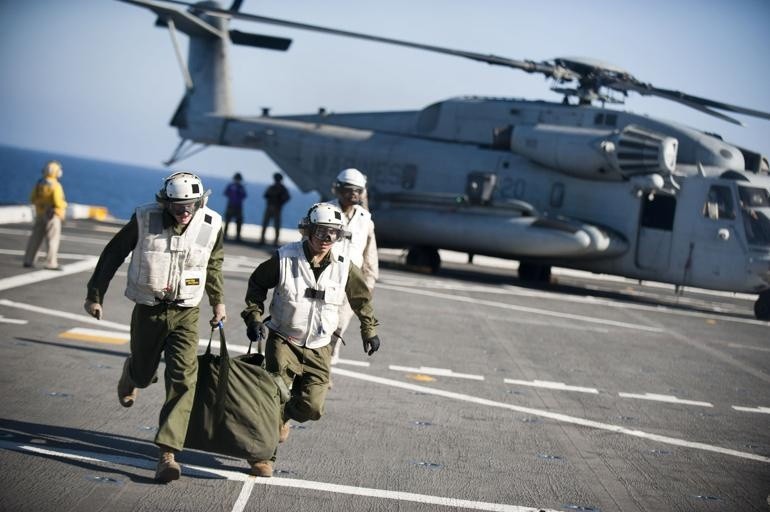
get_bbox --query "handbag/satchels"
[186,322,281,464]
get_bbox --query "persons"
[262,173,291,247]
[83,170,228,485]
[221,173,248,242]
[23,162,68,271]
[315,166,381,392]
[242,203,383,479]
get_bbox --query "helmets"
[333,169,367,201]
[43,162,61,177]
[299,204,344,242]
[156,173,209,210]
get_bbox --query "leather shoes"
[118,357,136,408]
[45,266,62,271]
[251,458,270,477]
[23,263,34,267]
[154,447,180,484]
[279,404,289,443]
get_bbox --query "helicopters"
[118,0,769,321]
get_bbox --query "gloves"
[247,323,265,341]
[363,336,380,356]
